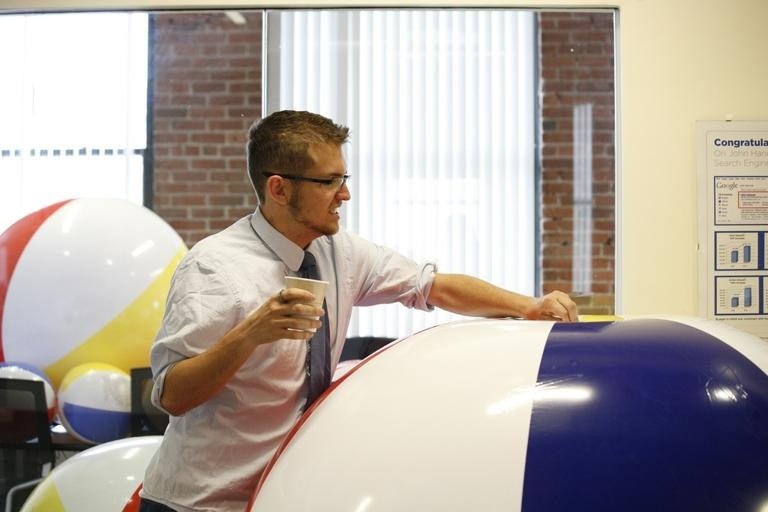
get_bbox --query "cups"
[283,275,326,336]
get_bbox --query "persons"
[138,110,578,512]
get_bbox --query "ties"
[300,252,332,413]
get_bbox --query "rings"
[279,289,285,302]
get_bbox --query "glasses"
[263,170,351,192]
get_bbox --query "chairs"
[128,367,171,437]
[0,375,56,512]
